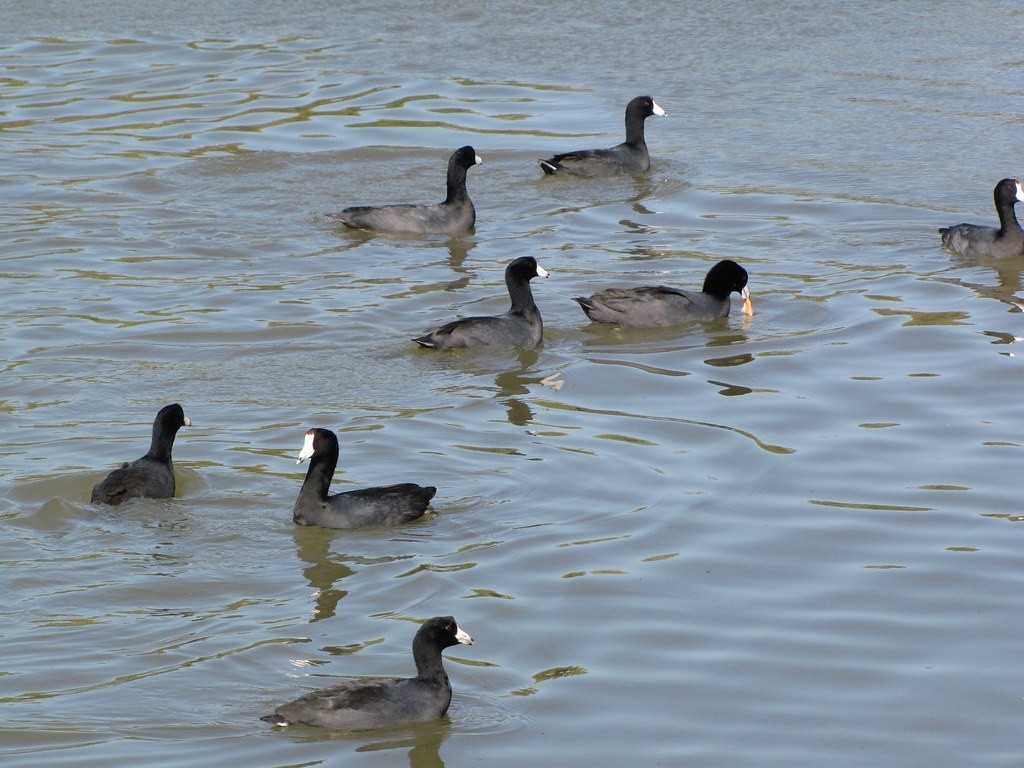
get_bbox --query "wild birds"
[536,91,670,183]
[293,428,436,529]
[260,615,474,732]
[408,257,549,360]
[91,404,191,507]
[920,178,1024,259]
[569,259,749,331]
[322,143,484,246]
